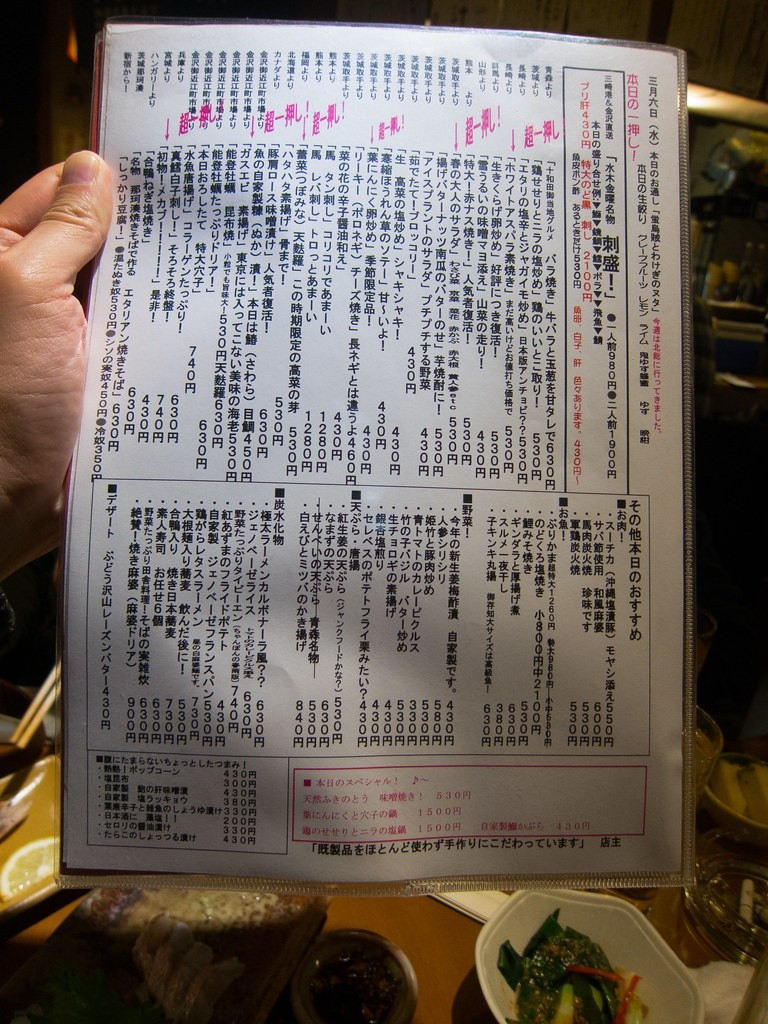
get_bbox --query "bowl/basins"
[291,928,419,1024]
[476,887,708,1024]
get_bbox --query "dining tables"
[0,753,768,1023]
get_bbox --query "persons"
[0,132,134,589]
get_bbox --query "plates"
[682,824,768,969]
[698,751,768,844]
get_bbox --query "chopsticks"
[9,659,64,748]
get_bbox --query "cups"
[578,704,724,912]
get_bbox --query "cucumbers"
[499,910,654,1024]
